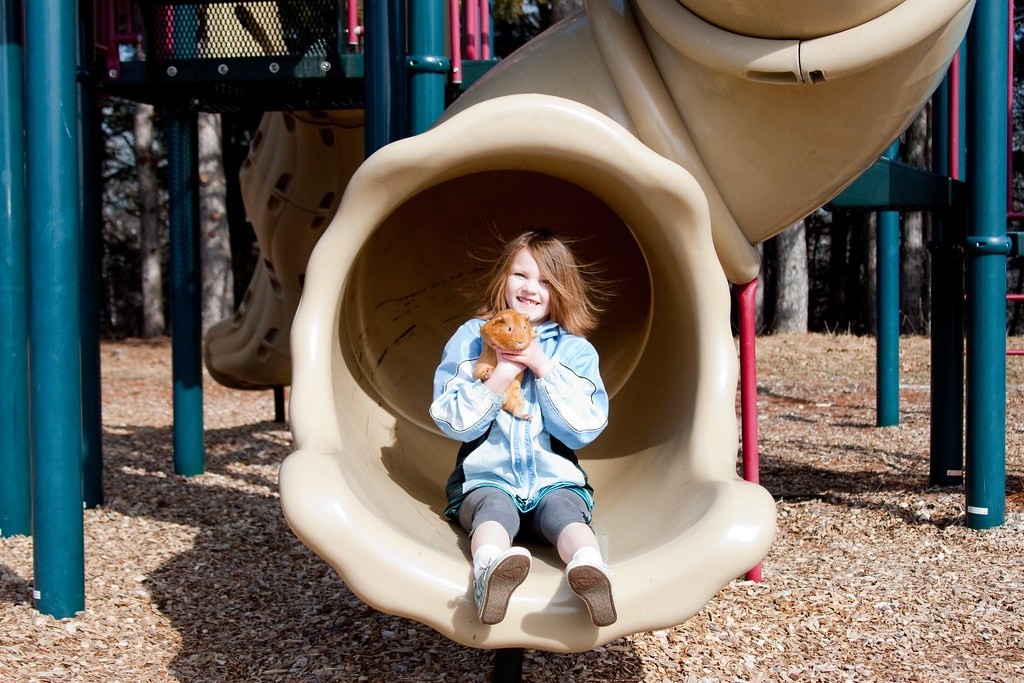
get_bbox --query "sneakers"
[472,546,533,625]
[565,557,618,627]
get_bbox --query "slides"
[276,0,982,655]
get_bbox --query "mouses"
[471,309,535,422]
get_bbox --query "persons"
[429,229,618,627]
